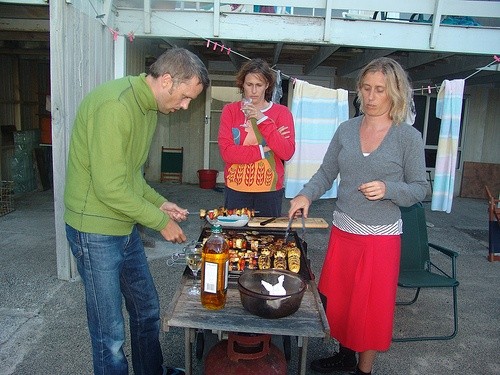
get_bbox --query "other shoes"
[166,367,185,375]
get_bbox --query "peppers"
[208,209,256,271]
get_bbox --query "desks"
[39,144,52,187]
[161,220,332,375]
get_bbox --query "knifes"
[259,217,277,225]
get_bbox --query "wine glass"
[239,98,253,128]
[185,243,202,295]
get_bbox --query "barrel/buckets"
[196,169,219,189]
[40,112,52,144]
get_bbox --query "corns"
[258,244,300,273]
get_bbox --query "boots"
[347,364,371,375]
[310,344,356,371]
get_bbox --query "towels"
[283,76,349,200]
[429,77,466,214]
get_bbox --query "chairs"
[391,202,460,343]
[484,184,500,262]
[160,146,184,185]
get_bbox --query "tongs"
[284,211,306,244]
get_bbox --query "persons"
[219,58,295,216]
[64,47,210,375]
[288,57,428,375]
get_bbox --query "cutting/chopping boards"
[247,216,329,228]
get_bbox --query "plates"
[206,215,250,227]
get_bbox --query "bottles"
[201,224,230,309]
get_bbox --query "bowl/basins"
[237,269,307,318]
